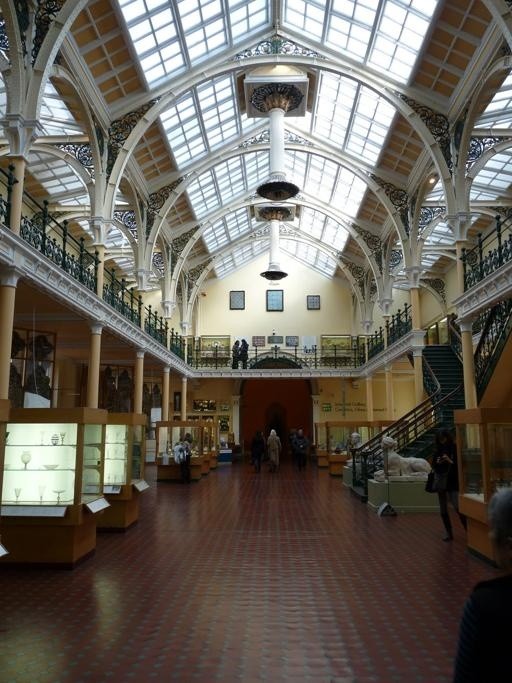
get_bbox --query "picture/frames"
[266,290,283,311]
[307,295,320,310]
[230,291,245,310]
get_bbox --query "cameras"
[437,456,445,463]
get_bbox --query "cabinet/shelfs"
[155,421,203,480]
[454,408,512,568]
[97,413,150,533]
[315,422,328,468]
[0,408,111,571]
[201,424,211,474]
[206,422,218,468]
[325,421,371,477]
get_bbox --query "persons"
[8,361,163,416]
[452,486,511,683]
[175,434,193,483]
[430,427,467,542]
[231,340,240,368]
[240,339,249,368]
[247,427,310,475]
[184,432,194,444]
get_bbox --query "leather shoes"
[442,536,454,542]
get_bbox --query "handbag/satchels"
[240,350,248,361]
[424,469,439,493]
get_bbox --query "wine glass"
[59,432,66,444]
[13,488,21,501]
[20,449,31,471]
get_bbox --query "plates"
[42,464,58,469]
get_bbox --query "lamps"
[235,63,320,201]
[246,202,302,280]
